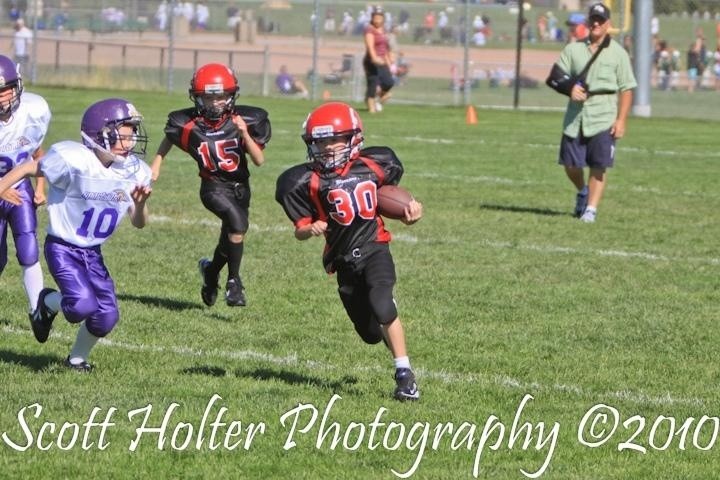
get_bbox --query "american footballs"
[377,185,412,219]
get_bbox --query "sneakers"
[63,356,96,372]
[199,257,217,306]
[29,287,59,343]
[573,184,589,216]
[583,209,596,224]
[225,278,245,307]
[394,367,419,401]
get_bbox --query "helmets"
[188,63,240,119]
[82,98,149,155]
[0,54,24,121]
[302,101,363,174]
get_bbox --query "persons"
[0,98,152,371]
[274,103,423,400]
[149,62,271,306]
[0,55,51,313]
[545,4,637,224]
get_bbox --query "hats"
[587,2,610,22]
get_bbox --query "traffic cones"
[464,105,478,127]
[322,90,329,100]
